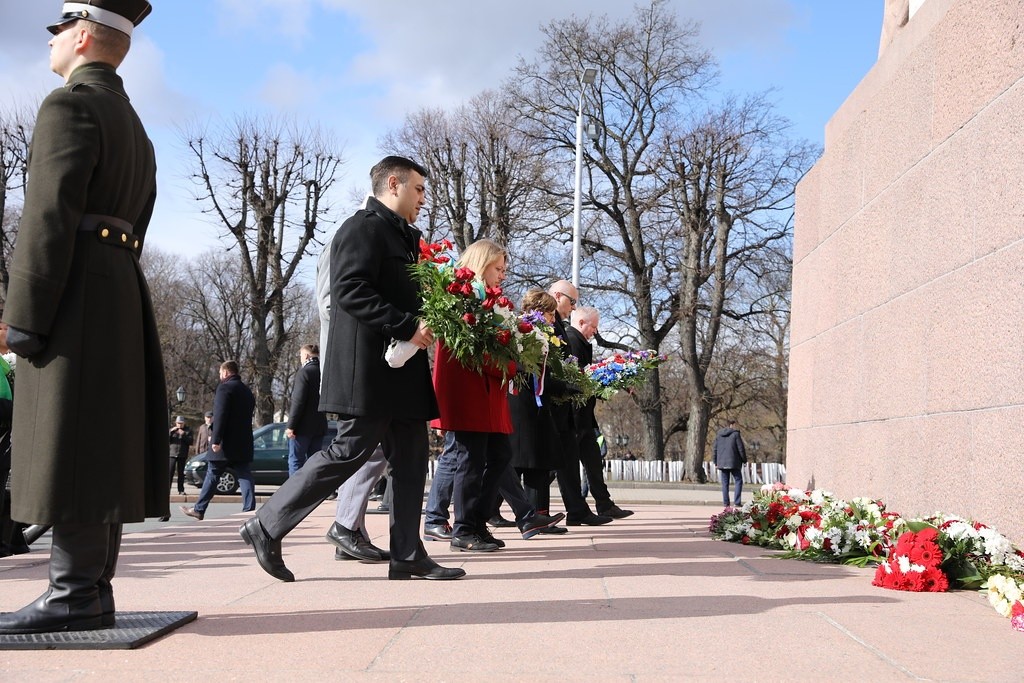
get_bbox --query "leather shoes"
[477,528,506,546]
[599,504,634,518]
[424,524,453,540]
[377,504,389,511]
[540,525,568,534]
[326,520,381,562]
[388,555,466,579]
[368,493,384,502]
[450,534,498,552]
[335,542,389,561]
[239,516,295,582]
[486,514,516,526]
[515,508,565,539]
[566,511,612,526]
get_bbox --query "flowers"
[399,234,671,407]
[706,482,1024,632]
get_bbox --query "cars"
[182,422,338,494]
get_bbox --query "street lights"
[176,385,186,414]
[571,67,597,323]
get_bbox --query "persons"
[242,156,466,581]
[0,0,174,632]
[168,415,193,496]
[421,238,634,553]
[179,360,255,521]
[193,410,215,457]
[711,417,749,508]
[285,343,328,479]
[323,160,390,563]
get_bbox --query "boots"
[0,521,122,632]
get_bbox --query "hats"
[175,416,185,423]
[205,411,212,417]
[47,0,152,37]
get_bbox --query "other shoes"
[179,491,186,495]
[734,503,743,507]
[179,504,202,520]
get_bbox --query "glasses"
[561,292,576,306]
